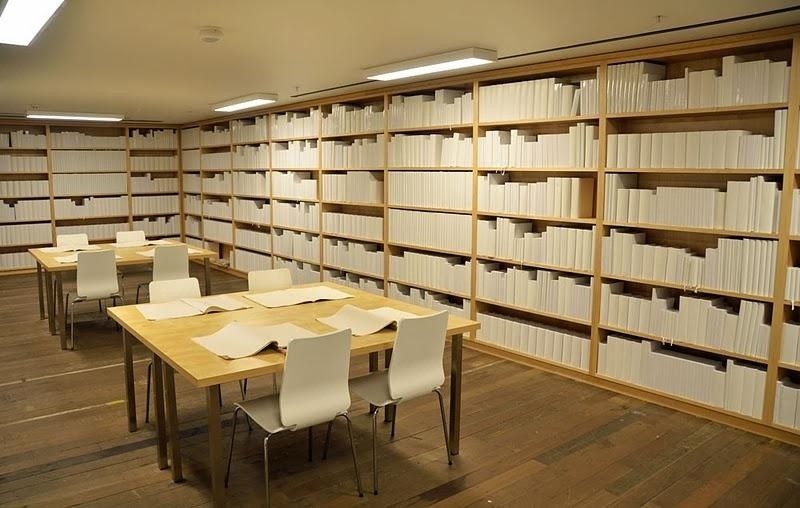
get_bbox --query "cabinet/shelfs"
[179,100,323,282]
[124,122,185,244]
[46,121,128,246]
[1,118,53,277]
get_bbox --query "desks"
[106,281,482,508]
[26,240,220,335]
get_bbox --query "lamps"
[1,1,68,48]
[209,92,280,114]
[24,108,123,122]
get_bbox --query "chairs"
[225,326,366,507]
[116,231,146,244]
[135,244,190,306]
[244,268,292,399]
[53,234,102,316]
[144,278,224,424]
[345,309,454,496]
[65,250,127,351]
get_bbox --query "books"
[1,105,320,288]
[476,67,600,371]
[245,284,354,308]
[190,322,319,360]
[315,103,384,297]
[387,88,472,335]
[135,293,251,324]
[598,54,800,428]
